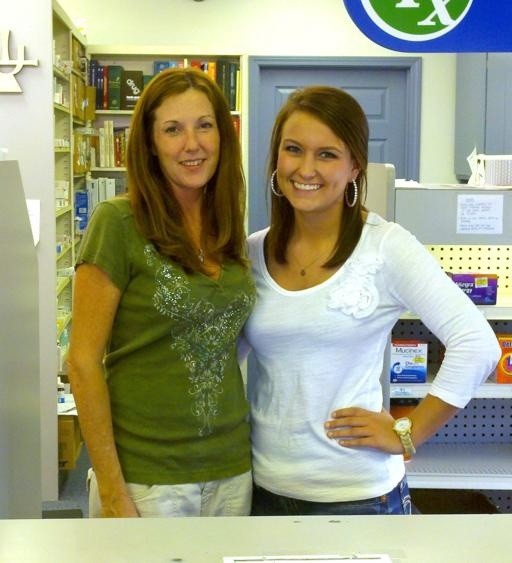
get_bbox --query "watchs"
[392,415,422,458]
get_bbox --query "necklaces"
[187,238,221,269]
[287,246,335,277]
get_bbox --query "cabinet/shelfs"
[0,0,88,501]
[360,162,512,491]
[89,44,249,237]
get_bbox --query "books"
[85,171,127,208]
[88,118,131,166]
[88,53,240,110]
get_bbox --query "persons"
[65,69,257,516]
[237,87,502,513]
